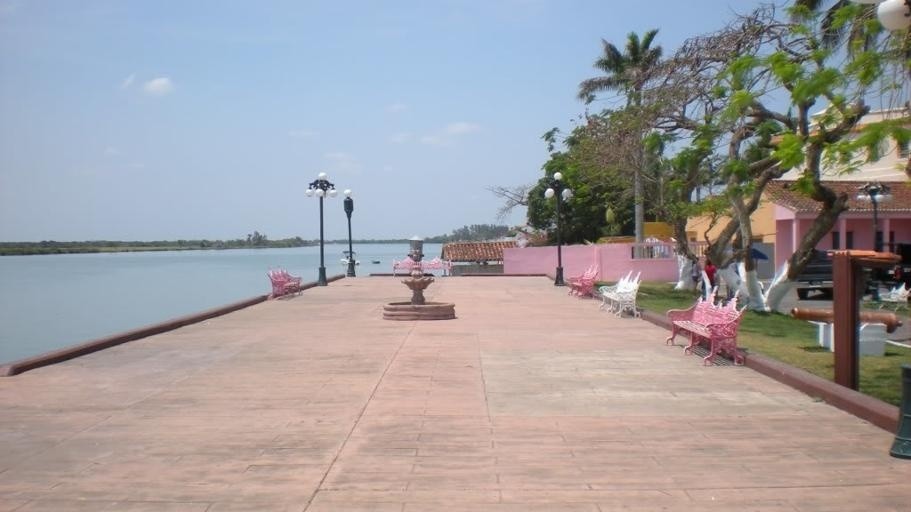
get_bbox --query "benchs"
[879,283,911,312]
[599,270,642,319]
[566,265,599,298]
[666,286,747,366]
[392,255,453,277]
[266,268,302,301]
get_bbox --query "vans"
[872,239,910,305]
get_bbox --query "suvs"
[787,249,876,300]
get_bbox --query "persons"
[691,258,717,289]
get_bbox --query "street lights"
[306,171,339,287]
[545,172,575,286]
[877,0,910,32]
[855,180,895,302]
[343,188,357,278]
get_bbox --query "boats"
[341,251,360,266]
